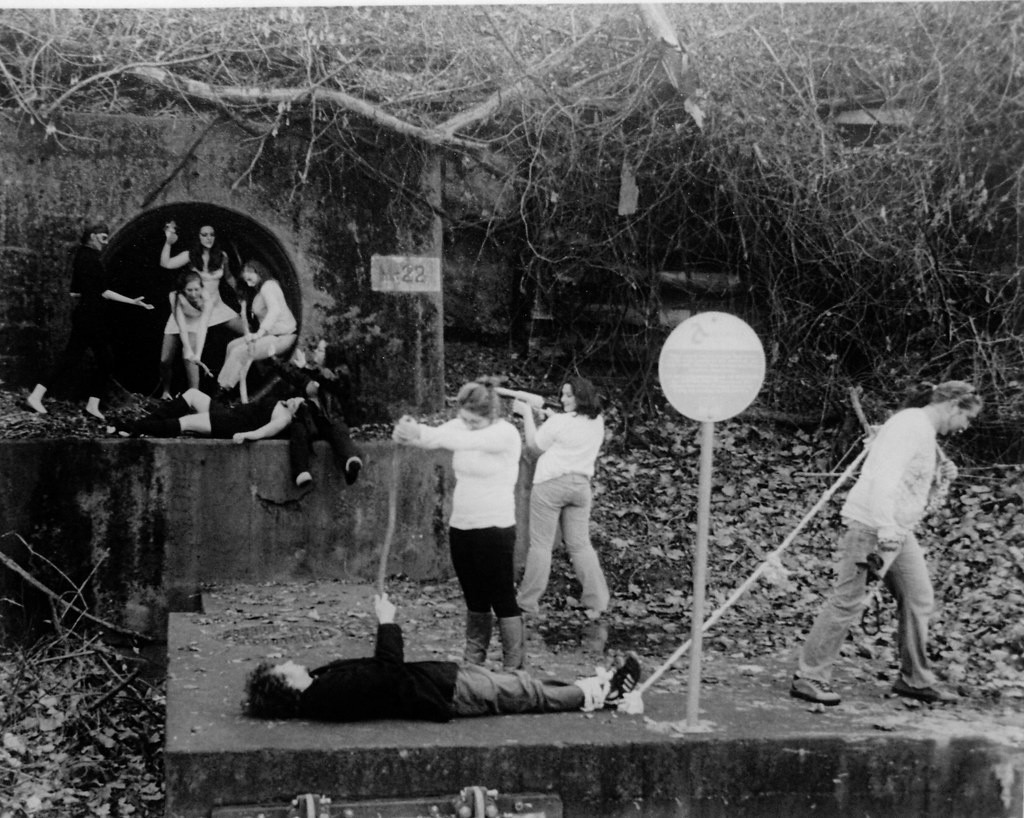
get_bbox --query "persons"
[512,376,611,623]
[242,592,641,720]
[25,223,155,420]
[790,380,982,704]
[110,217,362,494]
[393,375,524,672]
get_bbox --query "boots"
[497,607,525,673]
[461,609,492,666]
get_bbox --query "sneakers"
[790,674,841,706]
[893,677,957,704]
[602,655,641,709]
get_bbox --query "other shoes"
[344,457,361,485]
[570,602,588,610]
[21,399,52,417]
[295,471,313,487]
[83,409,105,420]
